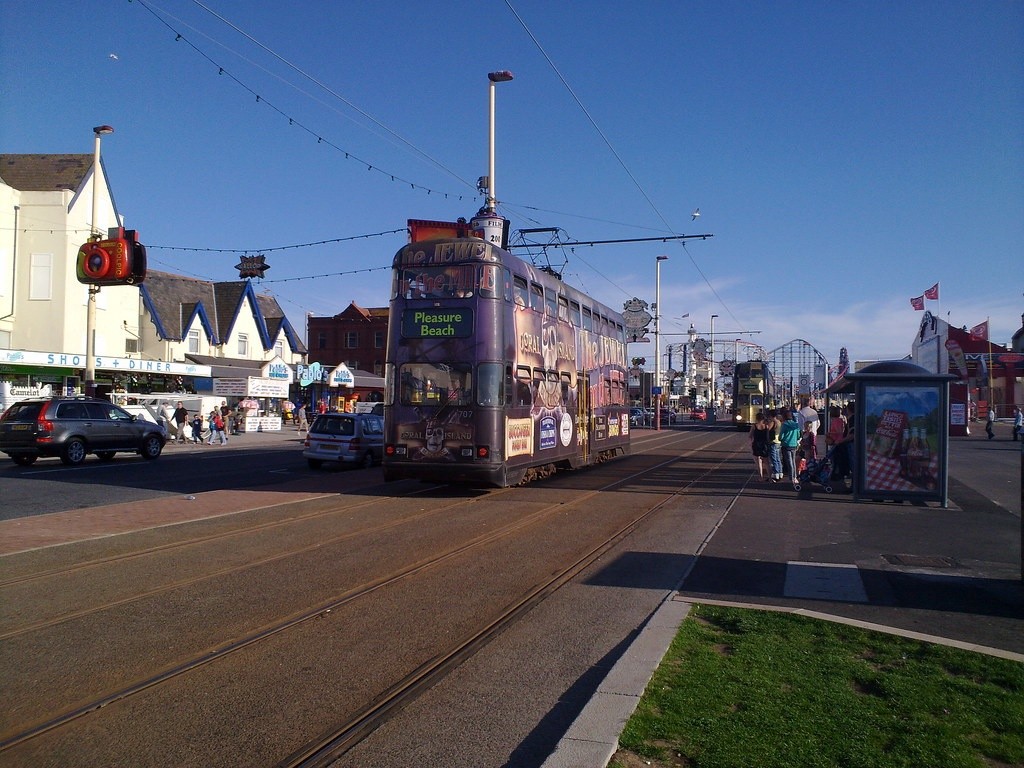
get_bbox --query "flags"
[910,283,939,311]
[969,320,987,340]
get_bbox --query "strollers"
[793,441,839,495]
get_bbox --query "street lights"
[486,69,514,212]
[654,254,668,432]
[83,125,116,402]
[710,314,719,410]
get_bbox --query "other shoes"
[207,442,212,446]
[770,474,780,480]
[776,472,783,479]
[220,444,226,446]
[784,480,794,484]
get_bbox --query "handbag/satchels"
[768,421,775,441]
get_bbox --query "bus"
[381,226,632,489]
[731,358,777,431]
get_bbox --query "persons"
[454,290,464,298]
[985,406,995,440]
[748,399,820,483]
[410,288,424,299]
[172,398,203,445]
[1012,405,1023,441]
[298,403,310,436]
[207,401,232,446]
[319,402,326,415]
[970,399,976,417]
[157,402,171,438]
[825,401,855,449]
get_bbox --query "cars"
[689,408,707,420]
[658,408,677,424]
[634,407,655,423]
[630,409,646,427]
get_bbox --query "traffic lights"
[688,387,696,399]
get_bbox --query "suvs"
[302,412,384,472]
[0,395,167,468]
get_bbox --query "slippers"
[767,478,774,483]
[753,479,764,482]
